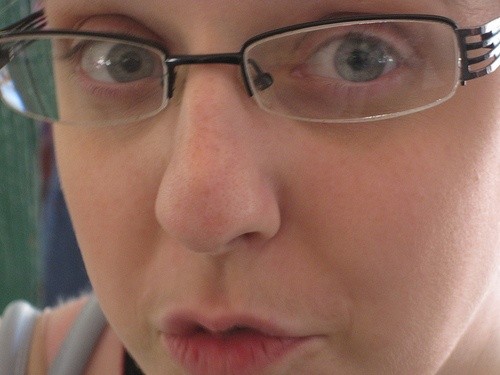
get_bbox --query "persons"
[0,1,499,374]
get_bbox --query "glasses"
[0,6,500,127]
